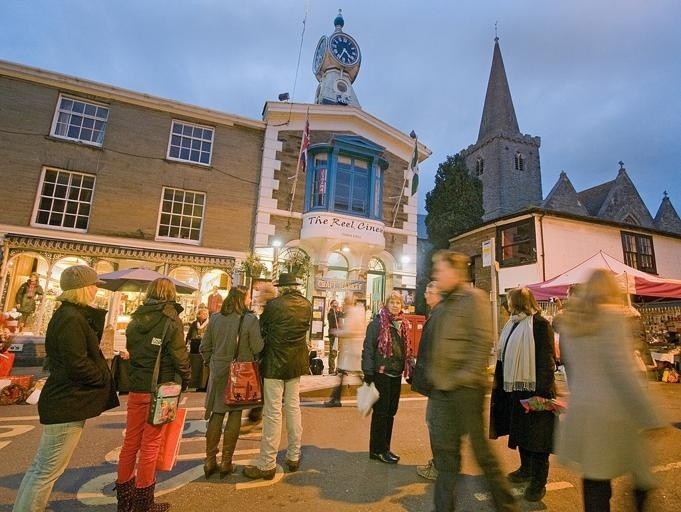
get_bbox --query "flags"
[300,114,310,171]
[404,143,420,197]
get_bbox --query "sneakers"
[417,461,440,480]
[330,368,334,374]
[324,400,342,407]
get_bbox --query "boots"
[509,447,535,482]
[113,474,135,511]
[129,479,171,512]
[524,460,550,501]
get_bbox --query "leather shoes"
[386,449,401,460]
[285,455,299,472]
[244,465,277,480]
[370,450,398,464]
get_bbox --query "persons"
[323,250,516,511]
[184,272,313,479]
[112,277,191,511]
[12,265,120,511]
[489,268,667,512]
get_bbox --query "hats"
[60,265,107,290]
[31,272,40,280]
[273,273,301,287]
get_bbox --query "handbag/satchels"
[148,382,183,425]
[357,383,380,417]
[225,361,265,405]
[136,407,187,471]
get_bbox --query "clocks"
[329,33,360,66]
[312,35,328,74]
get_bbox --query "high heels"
[204,463,220,479]
[220,462,237,479]
